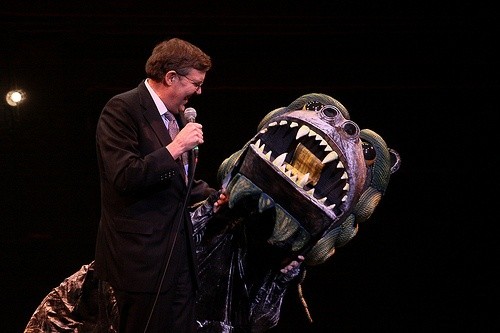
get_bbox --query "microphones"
[184,108,199,157]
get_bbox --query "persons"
[96,39,231,333]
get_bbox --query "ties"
[164,111,188,185]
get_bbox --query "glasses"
[172,70,203,90]
